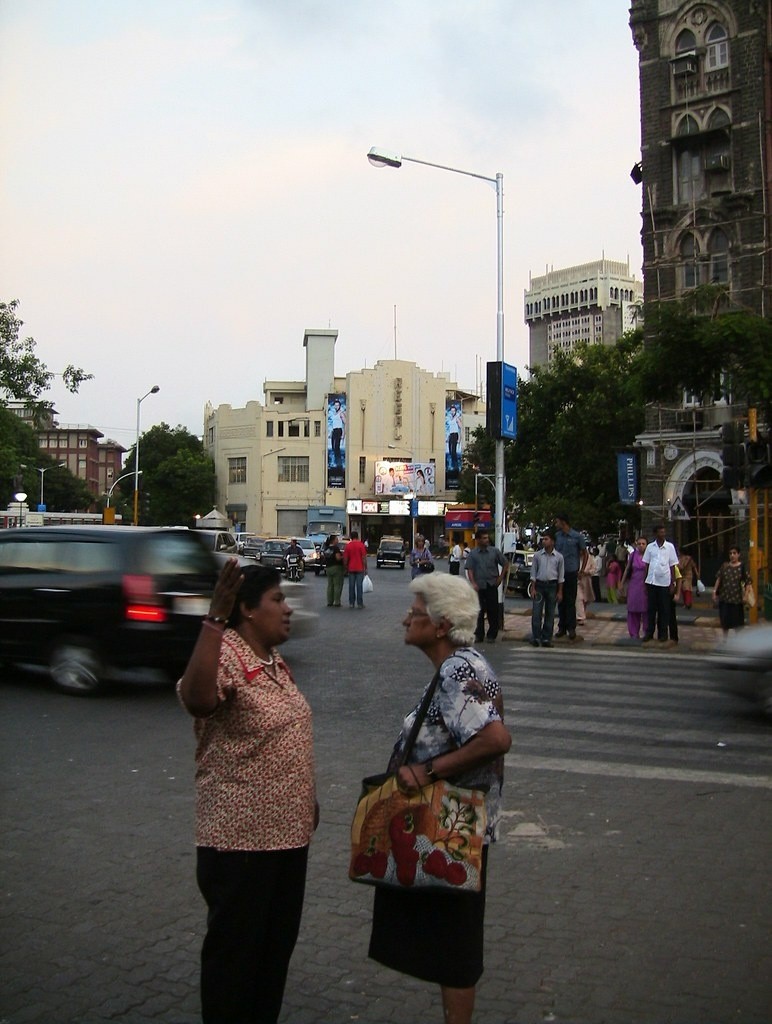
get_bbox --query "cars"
[375,539,407,570]
[313,538,351,575]
[231,528,317,570]
[208,550,322,646]
[0,523,236,697]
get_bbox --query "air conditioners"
[704,154,729,173]
[673,58,696,75]
[676,412,703,425]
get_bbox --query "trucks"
[303,505,347,548]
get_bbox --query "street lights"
[367,148,506,631]
[106,471,143,508]
[19,462,67,505]
[133,385,160,526]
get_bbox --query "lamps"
[631,161,643,184]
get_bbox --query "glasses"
[407,610,428,619]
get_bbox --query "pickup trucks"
[504,566,533,600]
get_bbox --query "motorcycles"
[283,554,307,583]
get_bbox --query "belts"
[536,580,557,585]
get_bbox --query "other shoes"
[350,603,355,608]
[529,639,539,646]
[569,630,576,640]
[541,640,550,647]
[358,605,366,609]
[327,604,343,607]
[555,630,567,638]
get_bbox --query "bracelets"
[200,613,230,635]
[423,760,438,781]
[712,590,716,593]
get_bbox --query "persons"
[283,538,305,579]
[446,406,464,472]
[176,558,320,1024]
[324,533,368,609]
[529,534,565,646]
[382,469,401,494]
[712,547,752,645]
[332,401,346,472]
[415,470,426,494]
[642,524,682,642]
[553,514,590,639]
[465,532,510,642]
[621,536,649,639]
[367,573,512,1024]
[410,534,470,580]
[515,538,701,625]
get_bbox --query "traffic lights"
[746,442,772,489]
[474,512,480,522]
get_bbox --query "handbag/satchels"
[349,774,488,892]
[362,575,373,593]
[744,585,756,608]
[419,562,434,573]
